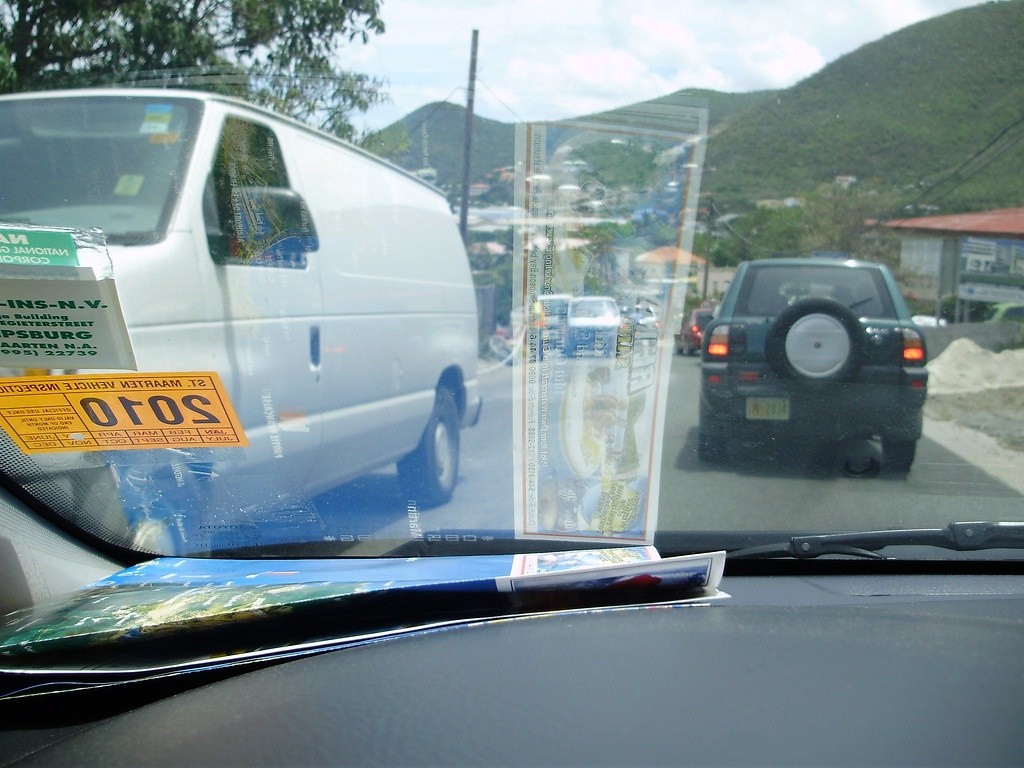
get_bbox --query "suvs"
[694,250,929,474]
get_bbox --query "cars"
[677,309,716,355]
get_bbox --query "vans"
[0,84,481,551]
[535,293,660,359]
